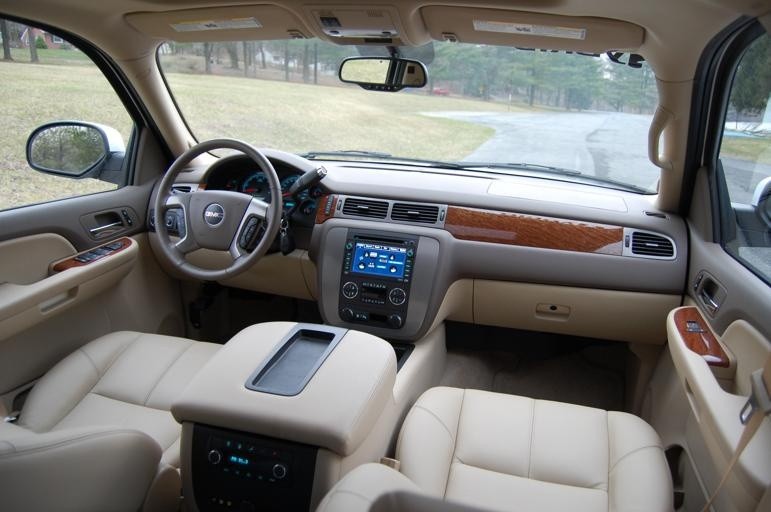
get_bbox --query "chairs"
[1,311,223,512]
[312,382,684,512]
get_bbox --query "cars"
[432,86,448,95]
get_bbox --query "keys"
[279,215,290,254]
